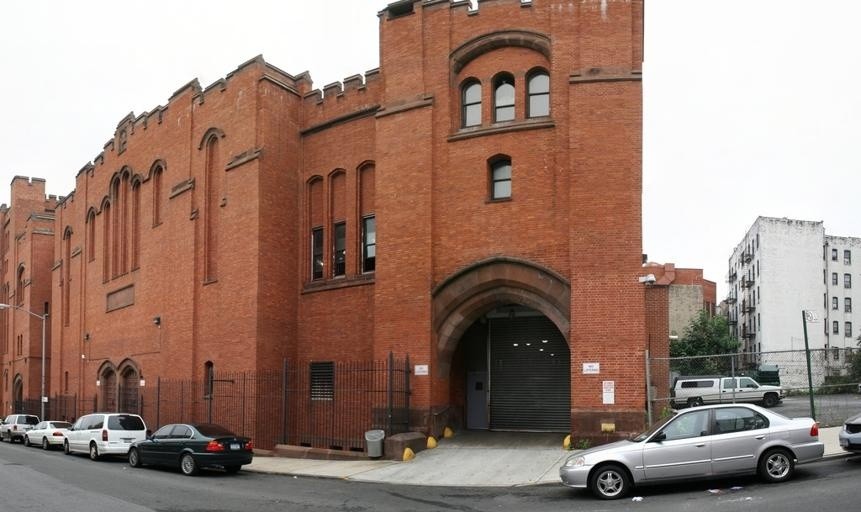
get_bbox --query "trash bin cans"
[365,430,385,457]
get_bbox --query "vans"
[60,412,148,462]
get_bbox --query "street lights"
[0,302,47,423]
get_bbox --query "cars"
[552,399,827,503]
[19,419,73,450]
[836,410,861,457]
[124,421,255,478]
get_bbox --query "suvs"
[0,413,40,445]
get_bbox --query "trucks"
[668,374,785,409]
